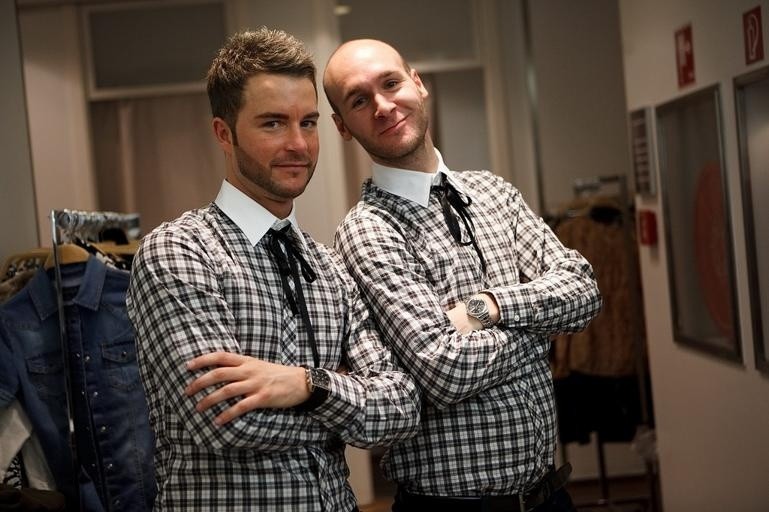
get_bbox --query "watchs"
[292,364,329,413]
[462,296,494,329]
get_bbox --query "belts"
[393,462,572,512]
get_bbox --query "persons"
[322,37,603,512]
[123,24,422,512]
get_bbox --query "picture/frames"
[654,83,743,370]
[732,64,769,373]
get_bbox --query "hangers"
[550,177,634,221]
[1,208,140,277]
